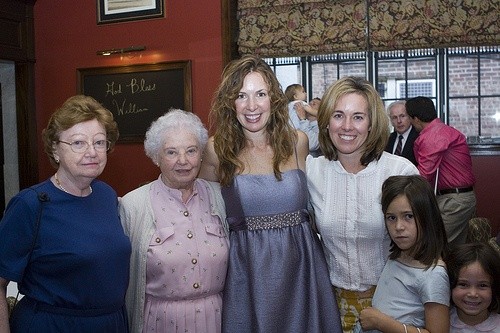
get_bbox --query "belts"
[437,186,473,195]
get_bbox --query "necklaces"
[54,173,93,194]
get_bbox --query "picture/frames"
[96,0,166,26]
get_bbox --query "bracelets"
[403,324,408,333]
[416,328,421,333]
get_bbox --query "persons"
[117,108,230,332]
[384,101,419,168]
[196,53,343,333]
[0,95,132,333]
[304,76,421,333]
[405,96,477,245]
[293,98,325,158]
[358,173,451,333]
[446,240,500,333]
[284,84,319,130]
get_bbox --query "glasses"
[57,139,111,153]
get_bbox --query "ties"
[394,134,403,155]
